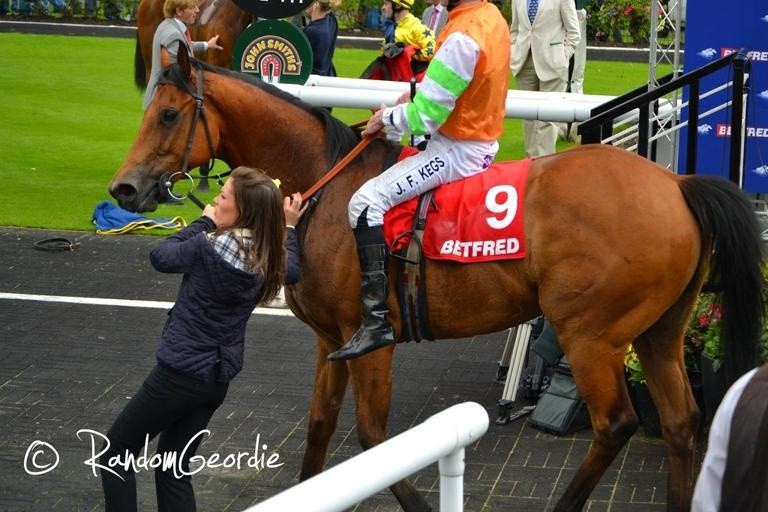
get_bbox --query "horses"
[107,39,768,512]
[133,0,254,94]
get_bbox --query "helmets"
[392,0,415,9]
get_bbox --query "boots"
[328,243,394,361]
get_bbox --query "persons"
[143,0,581,207]
[98,164,310,512]
[326,1,512,361]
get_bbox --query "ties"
[185,29,193,57]
[429,8,439,30]
[528,0,539,26]
